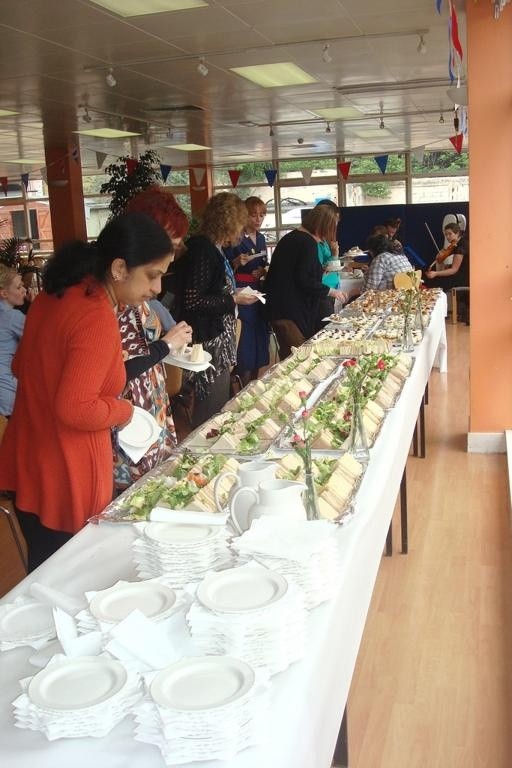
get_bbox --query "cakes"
[311,288,441,344]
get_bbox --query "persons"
[2,192,341,578]
[343,216,470,308]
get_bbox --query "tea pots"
[214,460,279,515]
[229,478,309,536]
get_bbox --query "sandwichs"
[183,453,241,511]
[277,351,411,451]
[276,452,362,521]
[181,339,337,449]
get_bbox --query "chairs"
[450,286,470,324]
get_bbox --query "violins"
[427,241,459,271]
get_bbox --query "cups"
[353,268,362,275]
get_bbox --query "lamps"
[258,108,460,137]
[84,28,430,87]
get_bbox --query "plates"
[145,521,221,588]
[0,599,66,641]
[150,656,254,713]
[345,252,361,257]
[324,266,345,271]
[170,347,212,365]
[28,657,127,713]
[117,406,160,449]
[196,567,287,613]
[88,583,177,623]
[253,293,267,296]
